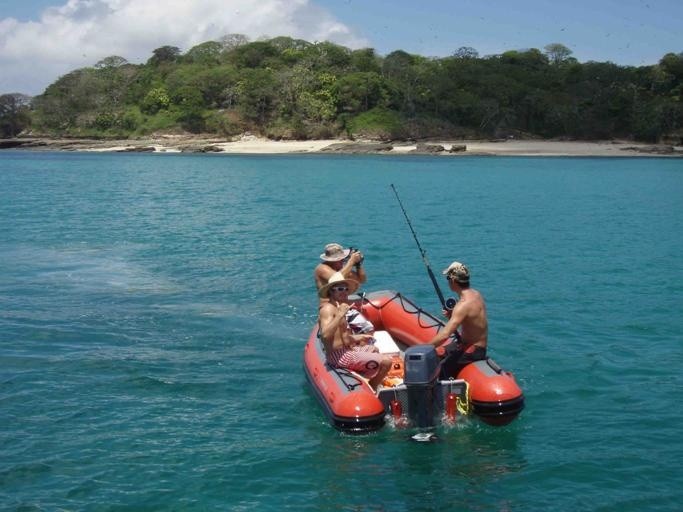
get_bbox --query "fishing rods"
[390,183,465,348]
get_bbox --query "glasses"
[332,285,350,293]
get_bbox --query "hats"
[318,243,351,263]
[317,271,359,299]
[441,260,471,284]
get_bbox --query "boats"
[302,284,526,436]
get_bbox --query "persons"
[315,243,375,338]
[319,272,392,395]
[424,261,487,382]
